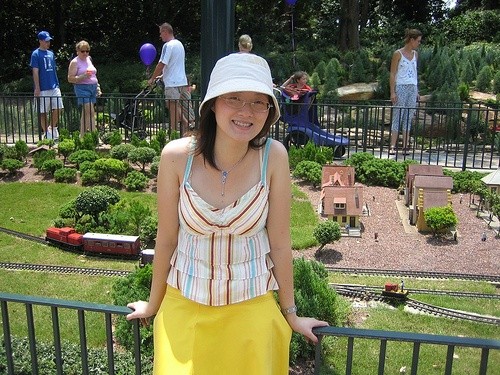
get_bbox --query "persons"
[282,70,316,102]
[67,39,103,141]
[29,31,64,141]
[237,34,253,55]
[388,29,422,156]
[146,22,195,132]
[126,54,330,375]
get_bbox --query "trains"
[381,284,410,301]
[44,225,156,265]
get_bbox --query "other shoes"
[183,124,195,136]
[403,147,410,155]
[98,138,104,145]
[389,146,396,155]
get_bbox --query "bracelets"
[281,304,298,315]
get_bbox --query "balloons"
[138,43,157,65]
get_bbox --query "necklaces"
[216,144,250,203]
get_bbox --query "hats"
[199,53,280,124]
[38,32,53,41]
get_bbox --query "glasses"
[216,96,273,112]
[80,50,89,53]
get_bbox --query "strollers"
[276,92,350,159]
[112,83,158,140]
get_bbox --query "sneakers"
[44,126,59,139]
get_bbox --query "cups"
[87,70,92,73]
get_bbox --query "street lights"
[282,12,296,70]
